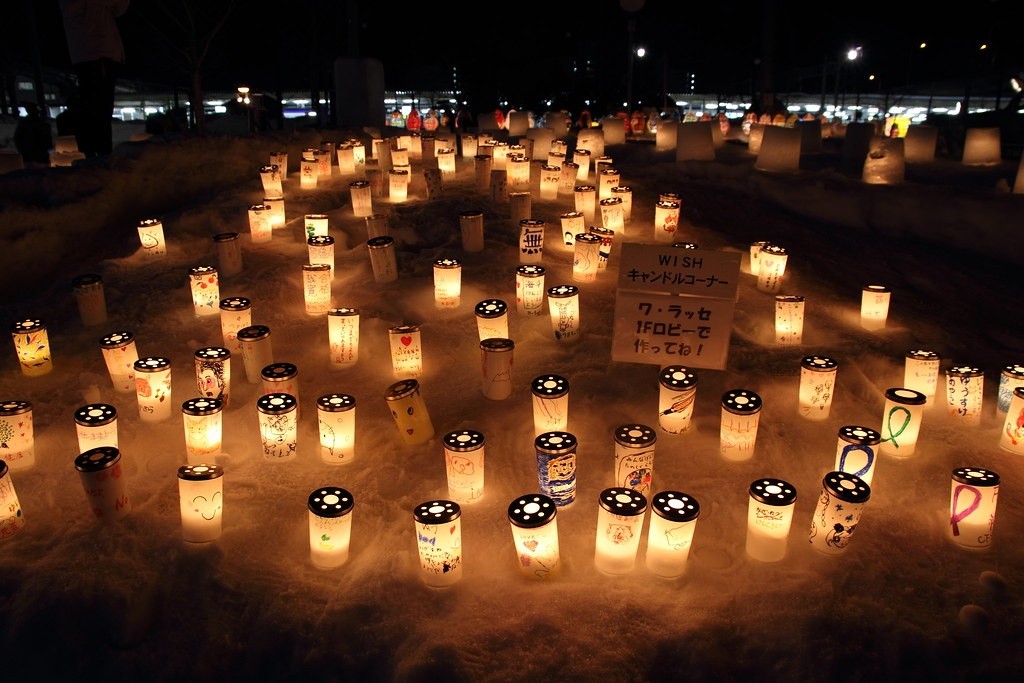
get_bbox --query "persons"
[62,0,129,166]
[13,103,52,169]
